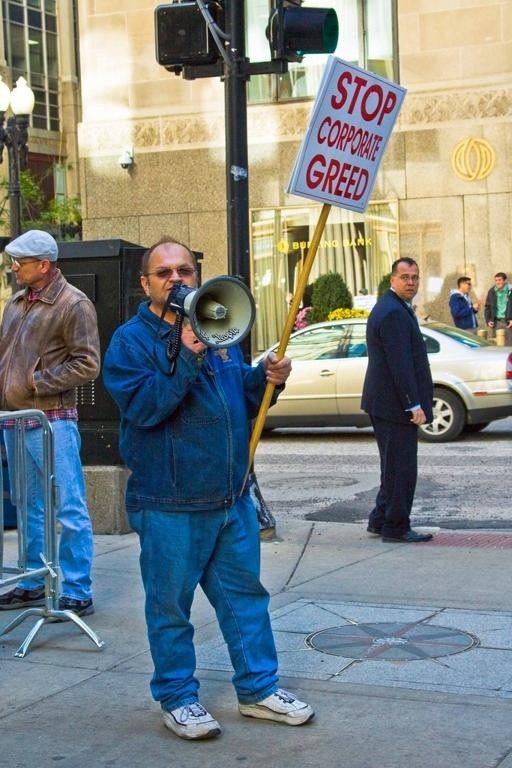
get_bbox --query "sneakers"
[46,591,96,623]
[160,698,223,741]
[0,587,47,610]
[238,687,315,726]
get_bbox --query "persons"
[0,229,103,621]
[450,275,480,334]
[361,257,436,542]
[484,272,512,347]
[102,238,317,742]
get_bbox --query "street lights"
[0,75,35,297]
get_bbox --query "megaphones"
[169,275,256,349]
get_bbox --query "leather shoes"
[381,526,433,542]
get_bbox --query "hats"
[4,229,59,265]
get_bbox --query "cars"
[253,314,512,444]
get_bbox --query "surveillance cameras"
[118,145,134,168]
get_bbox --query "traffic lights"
[265,1,339,61]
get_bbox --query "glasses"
[398,274,420,281]
[10,257,39,268]
[146,265,198,278]
